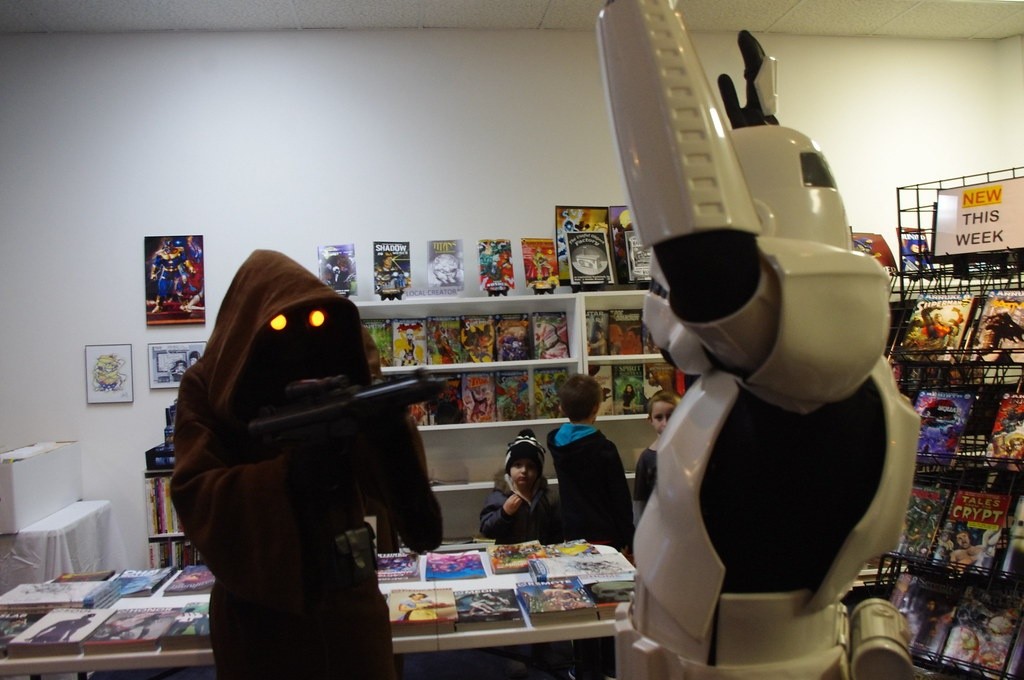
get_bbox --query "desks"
[0,542,636,680]
[0,499,111,592]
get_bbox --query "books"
[0,565,212,666]
[839,226,1024,680]
[371,540,636,638]
[145,401,206,568]
[313,203,693,426]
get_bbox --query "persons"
[595,0,922,680]
[479,429,552,543]
[542,373,635,552]
[163,249,441,680]
[633,390,682,527]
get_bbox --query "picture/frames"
[84,344,133,403]
[148,341,207,389]
[144,235,206,326]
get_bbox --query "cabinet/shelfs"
[849,166,1024,680]
[147,279,700,569]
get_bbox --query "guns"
[240,364,451,464]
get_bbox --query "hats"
[505,429,545,476]
